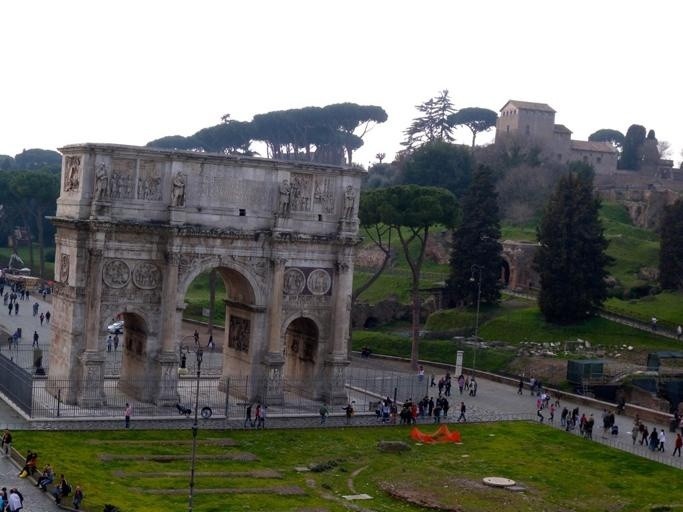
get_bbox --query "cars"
[106,319,123,335]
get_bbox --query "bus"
[1,263,40,293]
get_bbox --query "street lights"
[468,263,483,369]
[174,334,206,510]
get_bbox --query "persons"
[458,374,477,397]
[107,333,119,351]
[194,330,199,344]
[91,161,186,209]
[375,394,449,425]
[457,401,467,422]
[0,429,117,512]
[438,372,451,397]
[0,278,52,350]
[343,404,353,424]
[361,345,368,359]
[125,403,132,428]
[206,326,214,348]
[275,177,357,220]
[430,370,436,386]
[110,315,120,324]
[319,403,329,424]
[244,403,267,428]
[416,364,424,381]
[515,374,682,459]
